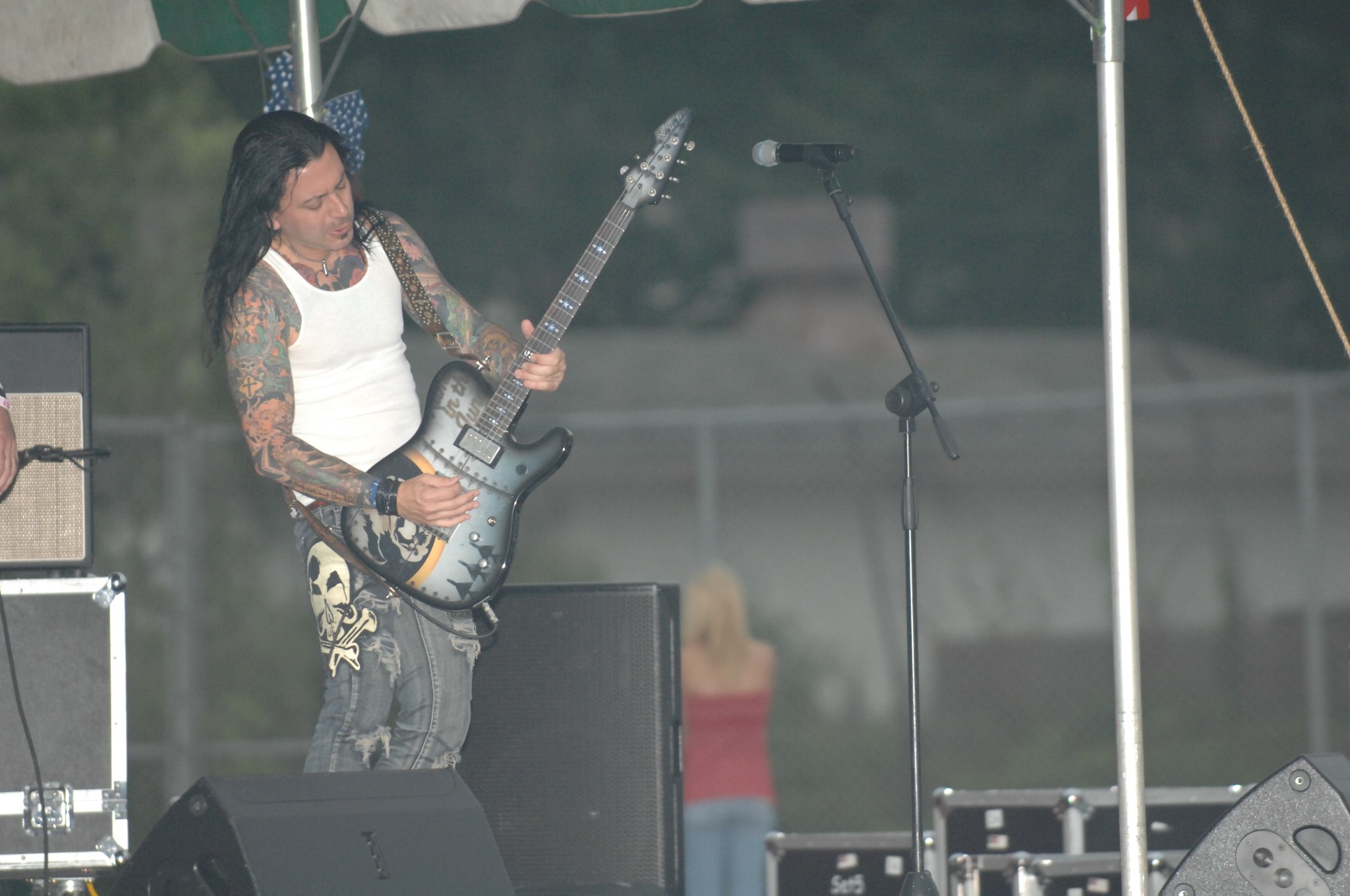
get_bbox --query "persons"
[680,564,778,896]
[200,110,567,769]
[0,385,18,497]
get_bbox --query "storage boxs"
[0,572,132,896]
[762,786,1250,895]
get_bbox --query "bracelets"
[0,396,9,413]
[375,475,405,517]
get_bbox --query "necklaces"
[277,234,331,278]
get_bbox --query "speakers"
[106,765,517,896]
[458,584,684,896]
[1155,753,1350,896]
[1,322,91,573]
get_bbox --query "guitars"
[340,103,702,616]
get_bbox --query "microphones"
[16,446,43,470]
[751,139,859,167]
